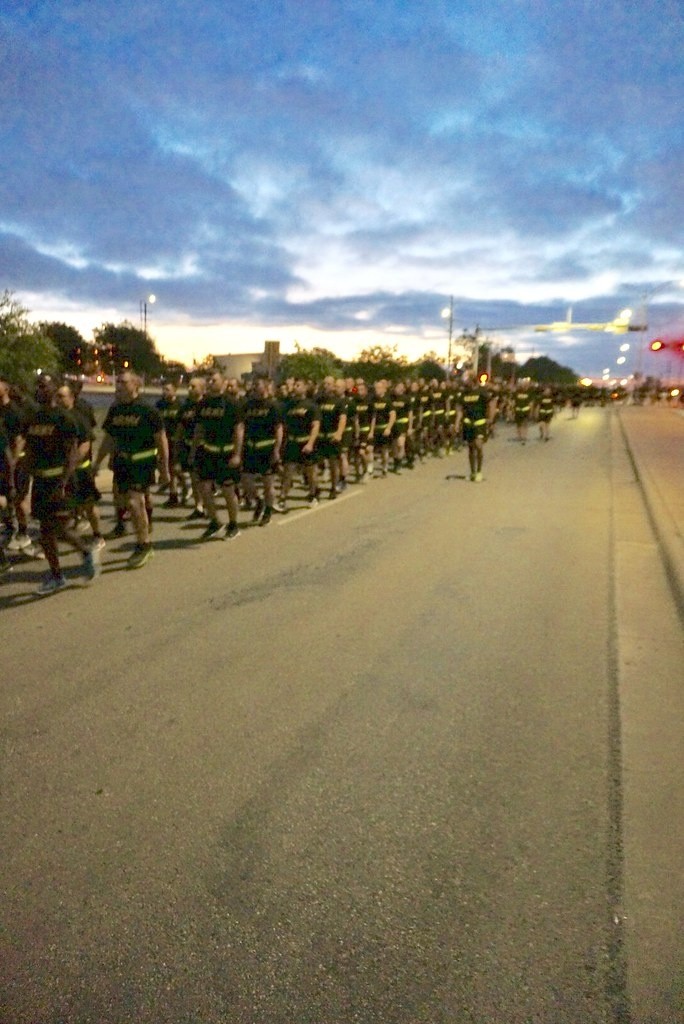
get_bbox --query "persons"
[0,371,684,595]
[535,389,554,442]
[454,371,495,482]
[508,380,533,445]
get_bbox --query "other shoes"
[7,533,31,550]
[105,520,128,541]
[475,472,482,482]
[77,534,104,581]
[465,472,475,481]
[127,544,155,568]
[1,562,14,576]
[35,573,66,595]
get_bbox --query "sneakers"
[155,458,415,541]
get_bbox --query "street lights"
[139,294,156,334]
